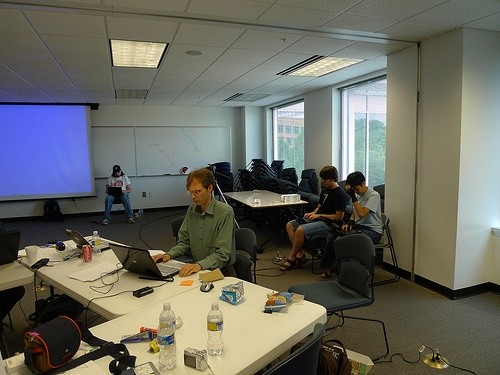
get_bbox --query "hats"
[113,165,121,172]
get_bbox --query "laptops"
[108,243,180,279]
[61,227,110,251]
[319,216,362,236]
[0,229,21,265]
[287,207,318,224]
[107,187,124,196]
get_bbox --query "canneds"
[82,245,92,262]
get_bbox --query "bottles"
[206,302,224,357]
[156,315,177,371]
[138,209,143,219]
[159,303,177,331]
[91,231,101,261]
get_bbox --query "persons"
[151,168,237,278]
[101,165,135,224]
[319,171,384,280]
[279,166,351,270]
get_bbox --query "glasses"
[187,187,206,196]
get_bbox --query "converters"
[133,285,154,297]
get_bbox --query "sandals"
[295,253,309,266]
[320,265,336,279]
[280,257,300,271]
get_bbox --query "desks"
[223,189,308,253]
[0,235,327,375]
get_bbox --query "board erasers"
[164,174,171,175]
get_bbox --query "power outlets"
[423,353,449,369]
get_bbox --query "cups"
[253,190,263,206]
[24,245,38,263]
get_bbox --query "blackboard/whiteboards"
[90,125,232,178]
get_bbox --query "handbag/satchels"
[317,338,352,375]
[24,316,81,375]
[34,294,83,324]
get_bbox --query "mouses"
[200,282,214,292]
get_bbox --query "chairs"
[288,234,389,362]
[171,159,401,287]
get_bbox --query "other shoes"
[103,218,111,225]
[128,217,135,223]
